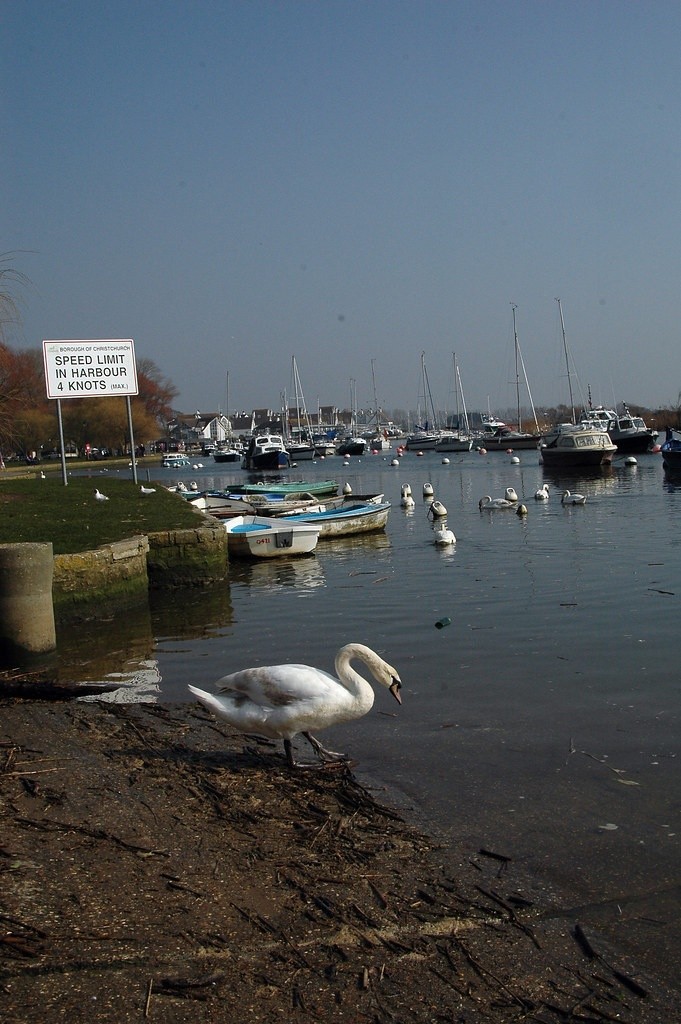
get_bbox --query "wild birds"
[140,485,156,494]
[93,488,110,502]
[40,471,46,479]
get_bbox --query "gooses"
[402,491,416,508]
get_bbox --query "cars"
[4,457,22,463]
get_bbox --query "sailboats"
[281,295,618,462]
[211,371,242,463]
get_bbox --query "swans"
[186,641,403,770]
[534,483,551,500]
[560,489,588,505]
[434,523,457,545]
[478,495,518,511]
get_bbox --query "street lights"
[167,419,176,453]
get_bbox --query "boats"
[229,442,244,454]
[224,514,320,556]
[539,421,619,469]
[602,401,659,453]
[161,453,190,466]
[241,435,289,469]
[659,428,681,465]
[169,480,391,537]
[202,445,216,454]
[228,554,326,591]
